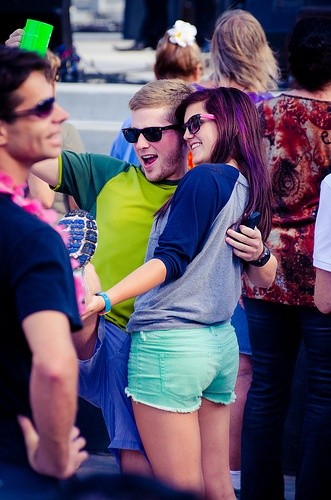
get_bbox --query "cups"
[19,19,54,59]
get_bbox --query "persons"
[0,46,84,499]
[183,9,281,471]
[110,21,205,174]
[78,86,273,499]
[5,28,277,500]
[239,18,331,499]
[312,173,331,315]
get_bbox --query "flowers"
[167,20,198,48]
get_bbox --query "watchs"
[95,290,112,317]
[246,242,271,267]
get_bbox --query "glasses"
[181,113,215,134]
[122,124,180,143]
[8,96,55,118]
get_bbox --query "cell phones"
[247,212,262,229]
